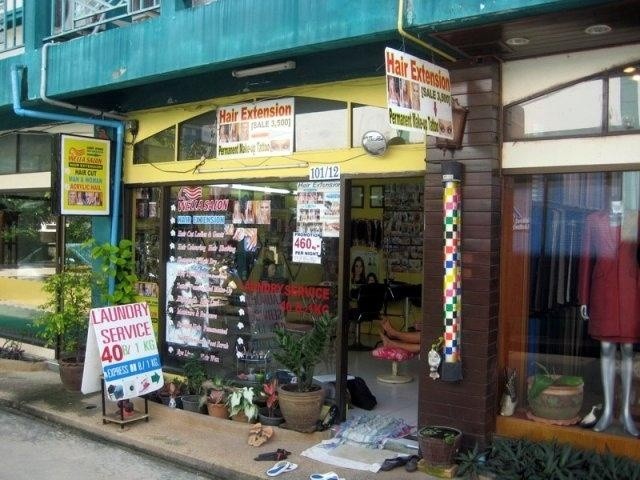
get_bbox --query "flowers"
[145,350,284,427]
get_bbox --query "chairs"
[349,282,386,351]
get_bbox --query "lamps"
[435,97,471,159]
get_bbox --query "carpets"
[300,437,415,473]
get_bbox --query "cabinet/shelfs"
[235,353,273,390]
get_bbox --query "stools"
[370,342,413,384]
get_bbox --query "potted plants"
[414,424,462,468]
[521,362,586,425]
[27,271,104,392]
[274,315,341,434]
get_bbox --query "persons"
[378,319,421,355]
[351,256,365,284]
[387,75,420,111]
[224,199,271,252]
[366,272,378,285]
[578,198,640,438]
[68,190,103,206]
[219,122,249,144]
[168,275,208,347]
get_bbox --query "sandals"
[247,421,274,447]
[380,455,409,473]
[255,449,291,462]
[405,454,422,473]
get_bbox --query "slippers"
[309,471,346,480]
[267,461,299,478]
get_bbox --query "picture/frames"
[369,184,384,208]
[351,184,364,209]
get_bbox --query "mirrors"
[361,129,388,157]
